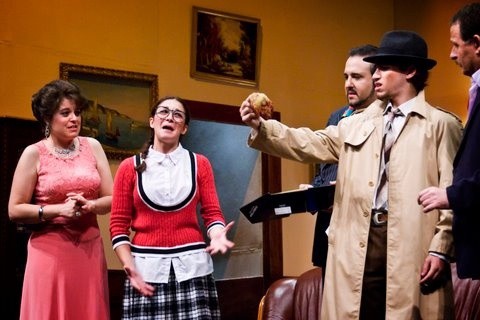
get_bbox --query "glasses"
[151,105,188,122]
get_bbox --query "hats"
[362,30,438,71]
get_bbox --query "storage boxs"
[239,184,336,224]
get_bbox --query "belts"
[368,210,388,227]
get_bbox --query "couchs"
[258,263,480,320]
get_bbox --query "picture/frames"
[59,62,159,160]
[190,6,261,90]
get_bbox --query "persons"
[300,44,378,268]
[109,97,236,320]
[7,80,114,320]
[418,3,480,281]
[240,31,462,320]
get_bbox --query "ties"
[380,109,397,183]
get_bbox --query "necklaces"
[54,144,75,153]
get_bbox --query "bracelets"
[38,205,43,222]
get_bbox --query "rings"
[76,211,81,216]
